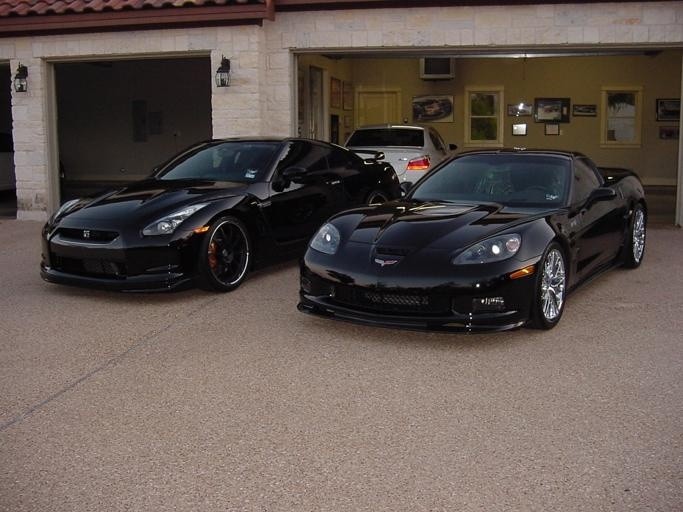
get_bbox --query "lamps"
[14,61,28,92]
[214,53,230,87]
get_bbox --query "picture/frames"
[658,126,679,143]
[507,98,597,136]
[655,98,680,122]
[410,92,454,123]
[327,76,354,146]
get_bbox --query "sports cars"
[292,144,651,336]
[37,132,403,295]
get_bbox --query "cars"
[343,122,459,195]
[413,97,453,122]
